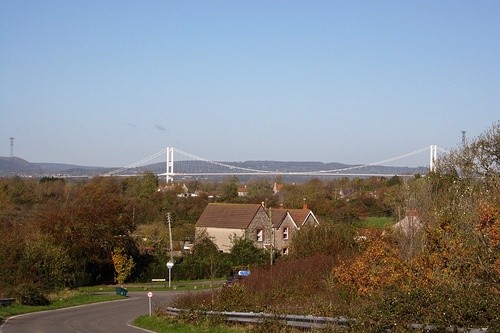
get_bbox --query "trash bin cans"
[115,287,128,296]
[0,297,16,306]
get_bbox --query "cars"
[223,276,239,288]
